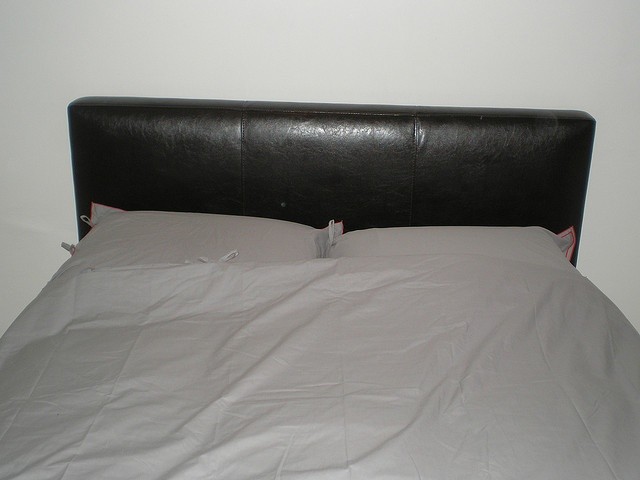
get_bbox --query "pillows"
[73,200,343,268]
[323,223,585,276]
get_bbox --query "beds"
[1,96,639,478]
